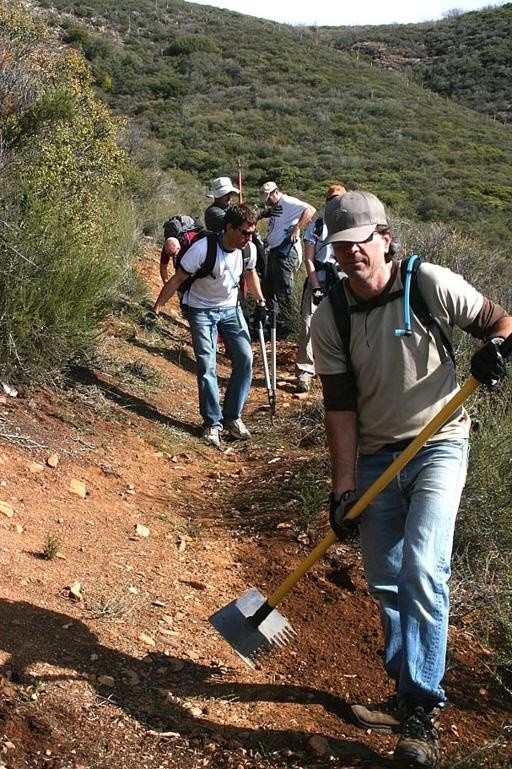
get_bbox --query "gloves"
[312,287,324,306]
[329,489,364,541]
[140,310,158,330]
[471,337,506,387]
[258,201,283,220]
[254,305,266,326]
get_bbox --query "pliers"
[257,310,276,415]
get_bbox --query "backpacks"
[163,214,204,238]
[174,230,250,319]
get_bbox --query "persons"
[312,190,511,764]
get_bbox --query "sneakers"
[223,418,252,439]
[199,428,220,446]
[348,694,443,769]
[294,372,311,393]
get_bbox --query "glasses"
[237,227,256,235]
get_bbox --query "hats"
[206,176,241,199]
[259,181,278,202]
[320,185,388,247]
